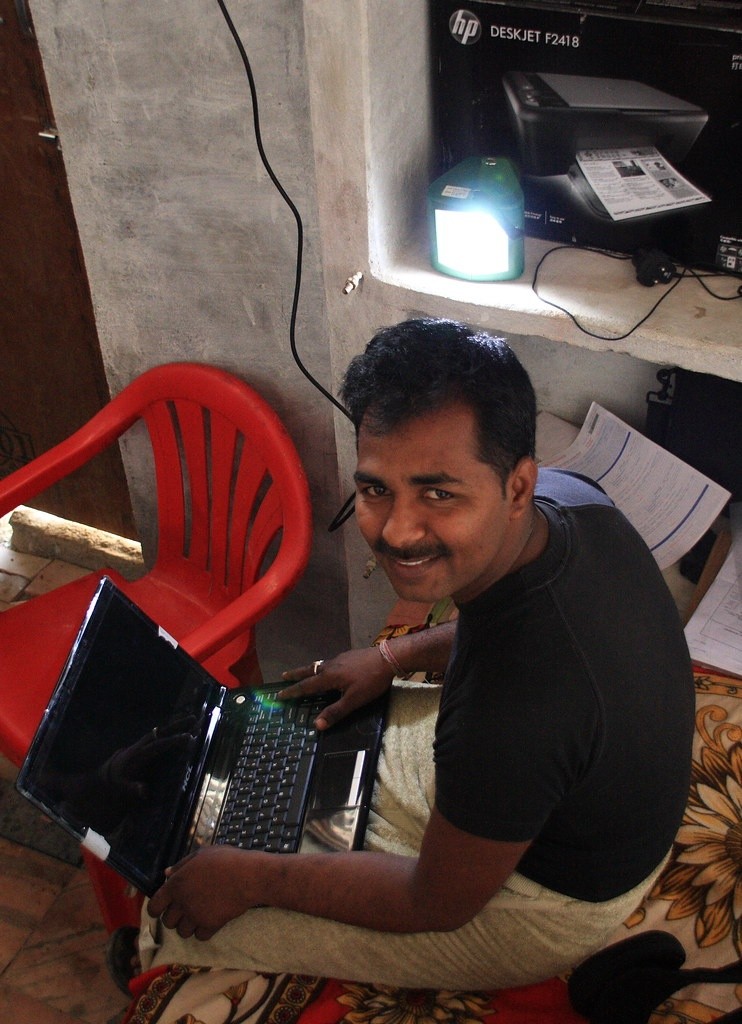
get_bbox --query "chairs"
[0,361,315,939]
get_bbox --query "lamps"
[426,156,526,283]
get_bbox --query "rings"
[313,660,324,675]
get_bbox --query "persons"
[105,317,696,1000]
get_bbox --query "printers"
[501,71,708,224]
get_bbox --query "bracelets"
[379,641,407,678]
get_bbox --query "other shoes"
[105,926,143,1000]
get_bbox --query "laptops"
[14,574,395,899]
